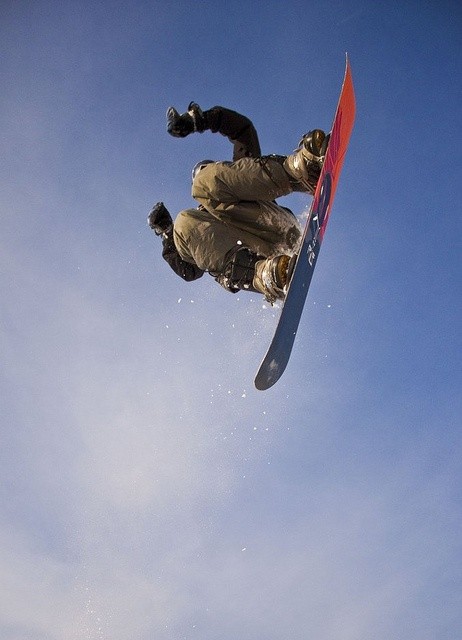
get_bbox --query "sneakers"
[253,255,290,299]
[290,129,326,194]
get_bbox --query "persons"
[147,101,328,303]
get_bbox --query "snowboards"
[254,53,356,392]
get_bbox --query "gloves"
[166,101,207,138]
[147,202,175,242]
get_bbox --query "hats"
[192,160,215,178]
